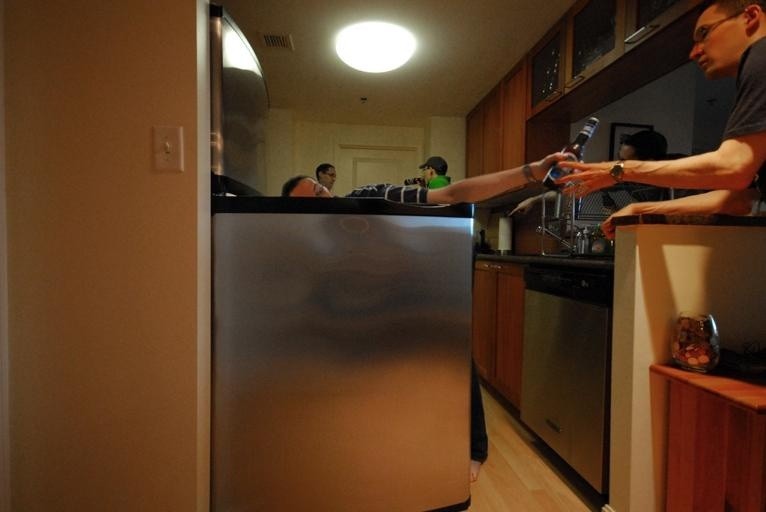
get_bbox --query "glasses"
[694,10,744,42]
[323,172,335,177]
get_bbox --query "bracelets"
[522,163,537,184]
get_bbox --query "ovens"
[511,266,610,498]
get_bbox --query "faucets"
[536,224,571,246]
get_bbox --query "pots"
[534,222,603,258]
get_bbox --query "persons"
[553,0,766,242]
[418,155,450,191]
[507,129,697,217]
[280,152,571,482]
[314,162,337,192]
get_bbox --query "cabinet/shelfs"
[624,0,703,54]
[466,66,525,198]
[473,259,525,411]
[527,1,624,122]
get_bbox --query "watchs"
[610,158,626,185]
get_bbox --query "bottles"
[670,310,722,372]
[540,117,600,198]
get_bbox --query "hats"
[420,157,447,172]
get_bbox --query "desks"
[650,360,765,512]
